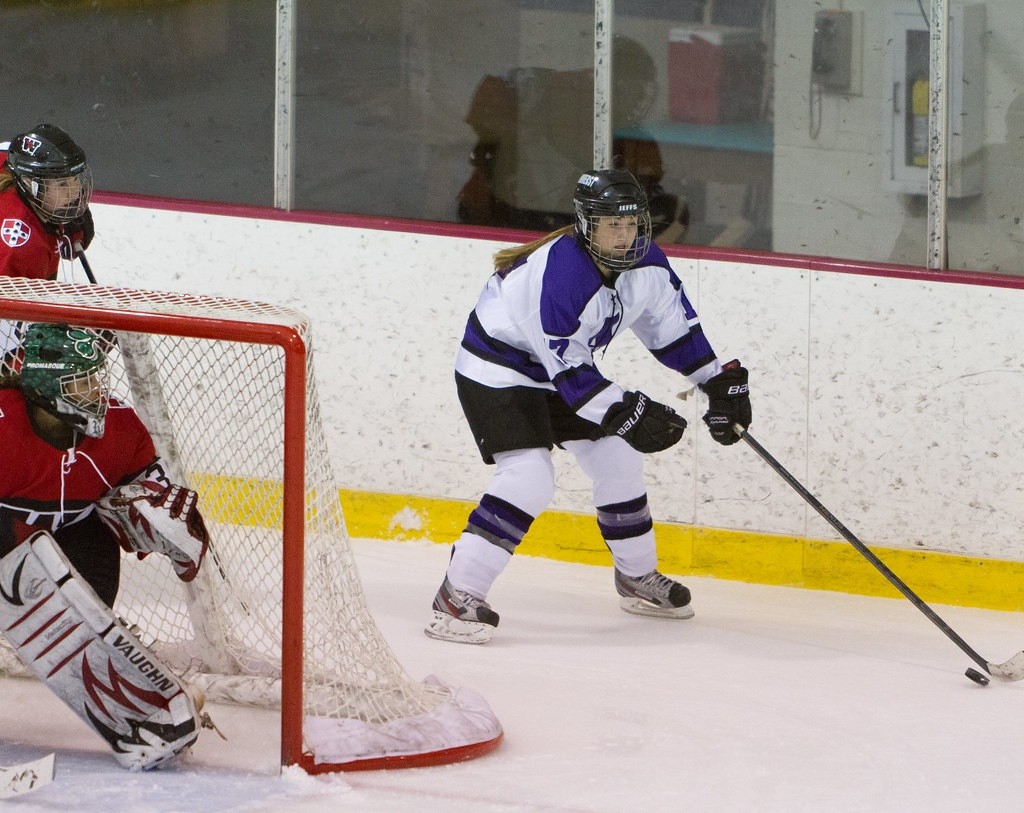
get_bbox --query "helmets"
[573,168,652,272]
[20,321,118,439]
[612,34,660,132]
[9,123,91,224]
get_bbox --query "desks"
[614,117,774,233]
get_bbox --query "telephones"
[809,11,852,90]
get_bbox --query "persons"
[0,318,216,775]
[1,124,96,280]
[453,26,688,234]
[422,167,757,647]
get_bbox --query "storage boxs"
[667,23,764,125]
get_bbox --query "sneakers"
[614,565,694,619]
[425,545,500,644]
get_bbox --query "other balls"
[964,668,990,687]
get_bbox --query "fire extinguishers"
[907,63,931,166]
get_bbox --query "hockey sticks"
[75,242,99,287]
[1,750,59,800]
[734,420,1024,682]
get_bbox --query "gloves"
[600,391,687,453]
[698,359,752,445]
[51,208,95,259]
